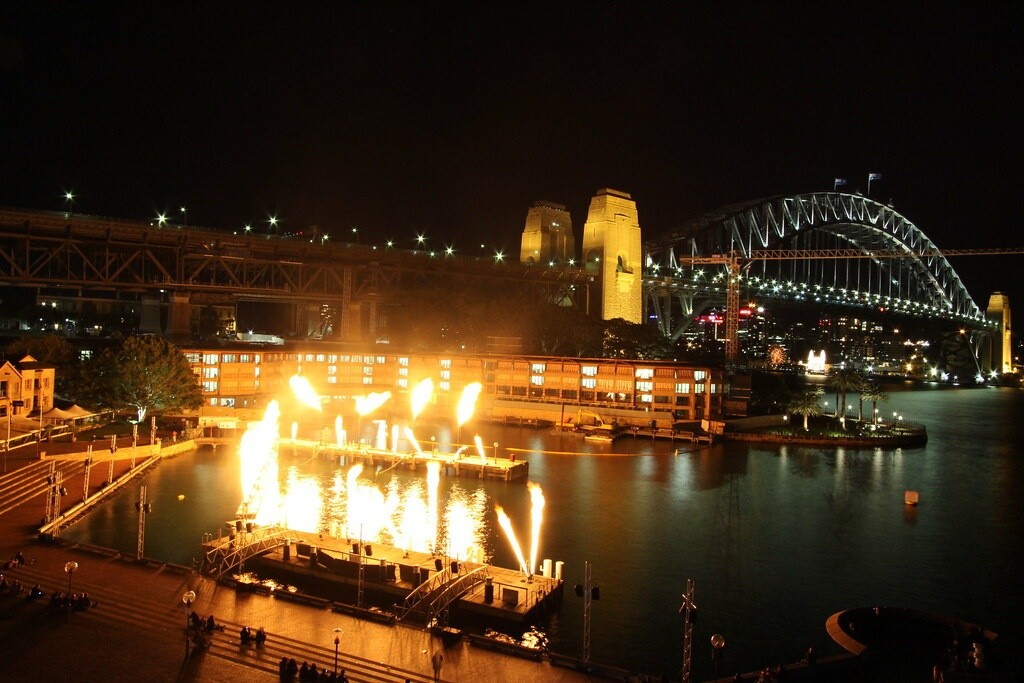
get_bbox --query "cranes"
[678,243,1024,395]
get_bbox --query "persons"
[0,579,23,594]
[207,614,226,631]
[430,650,443,682]
[50,590,98,609]
[256,627,266,645]
[7,552,25,569]
[190,611,206,627]
[31,585,45,598]
[172,431,177,442]
[278,656,349,683]
[240,626,254,644]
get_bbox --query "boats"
[823,603,1002,683]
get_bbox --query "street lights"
[332,628,343,678]
[874,407,880,426]
[823,401,828,416]
[270,217,279,240]
[876,416,882,438]
[892,411,897,435]
[847,404,853,438]
[182,590,197,659]
[64,560,79,625]
[180,206,188,228]
[352,228,359,246]
[709,632,726,683]
